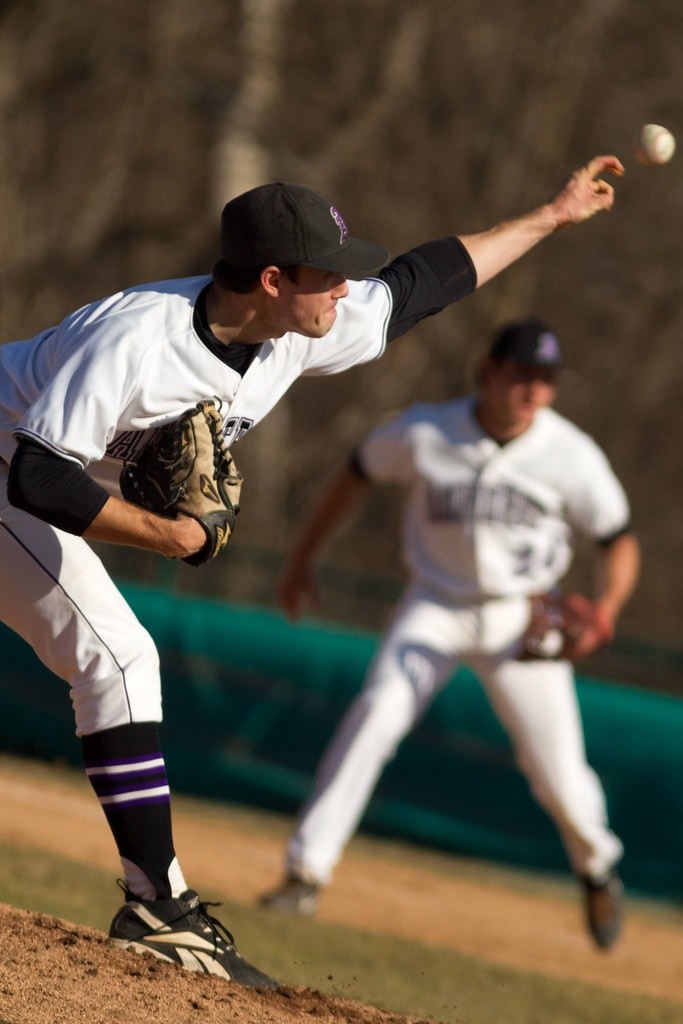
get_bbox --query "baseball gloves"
[119,399,242,567]
[514,591,615,662]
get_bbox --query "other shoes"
[577,858,625,949]
[258,883,321,913]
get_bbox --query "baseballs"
[634,123,676,167]
[531,627,566,659]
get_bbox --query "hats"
[217,182,390,276]
[488,318,561,377]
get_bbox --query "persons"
[254,317,644,955]
[0,152,627,998]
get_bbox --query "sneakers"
[107,877,279,992]
